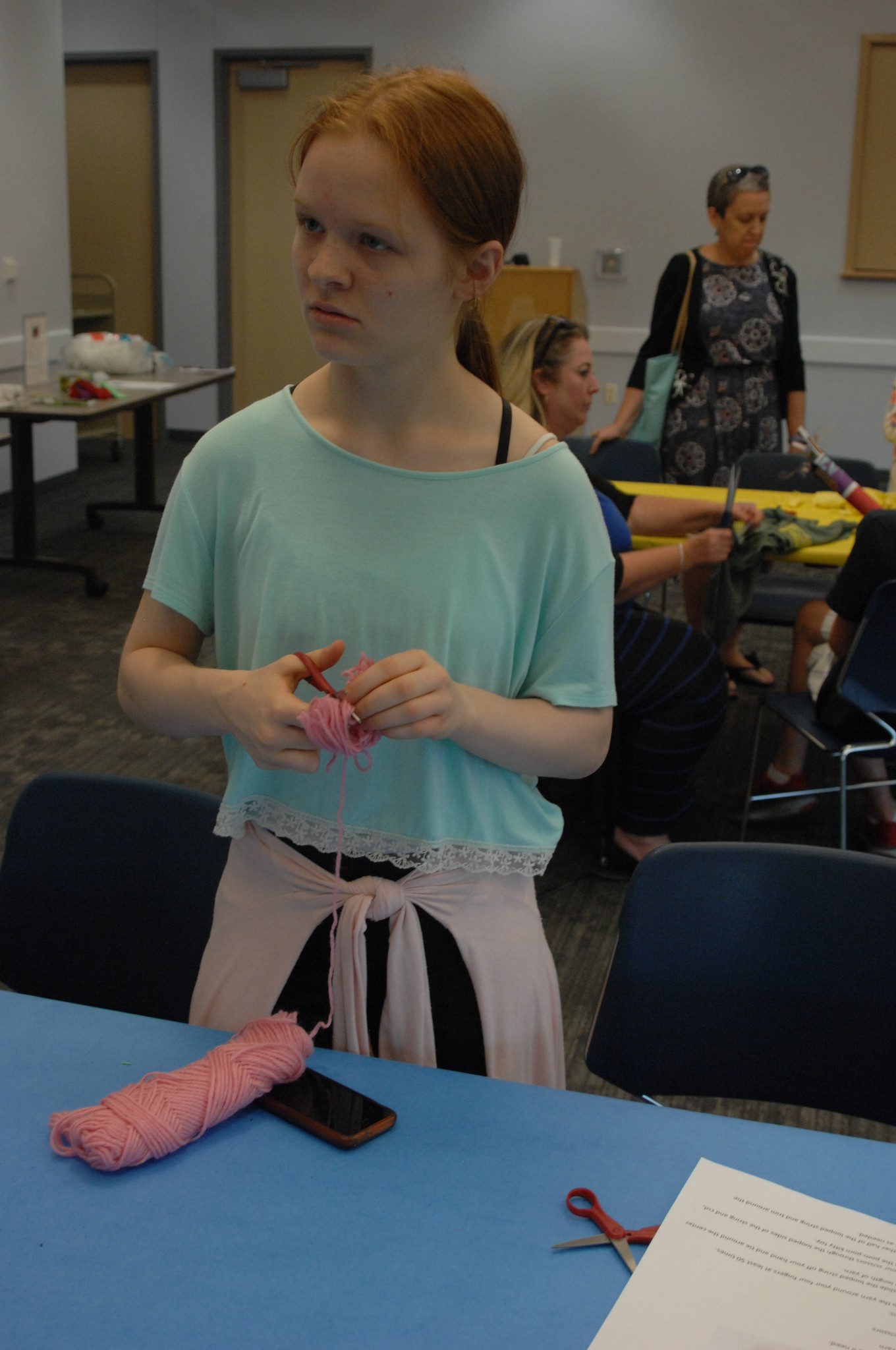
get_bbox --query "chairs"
[0,774,234,1024]
[589,843,896,1134]
[738,576,896,856]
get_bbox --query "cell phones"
[257,1066,398,1147]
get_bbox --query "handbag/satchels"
[625,251,697,451]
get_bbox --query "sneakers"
[851,819,895,856]
[739,768,817,819]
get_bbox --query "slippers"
[731,653,772,688]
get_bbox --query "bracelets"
[678,542,683,573]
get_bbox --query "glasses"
[721,166,769,190]
[536,315,571,365]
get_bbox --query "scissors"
[551,1187,661,1275]
[294,651,362,725]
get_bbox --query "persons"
[118,71,615,1092]
[493,316,763,879]
[588,166,809,695]
[745,511,896,860]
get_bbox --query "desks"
[1,366,239,591]
[608,479,886,566]
[0,988,896,1350]
